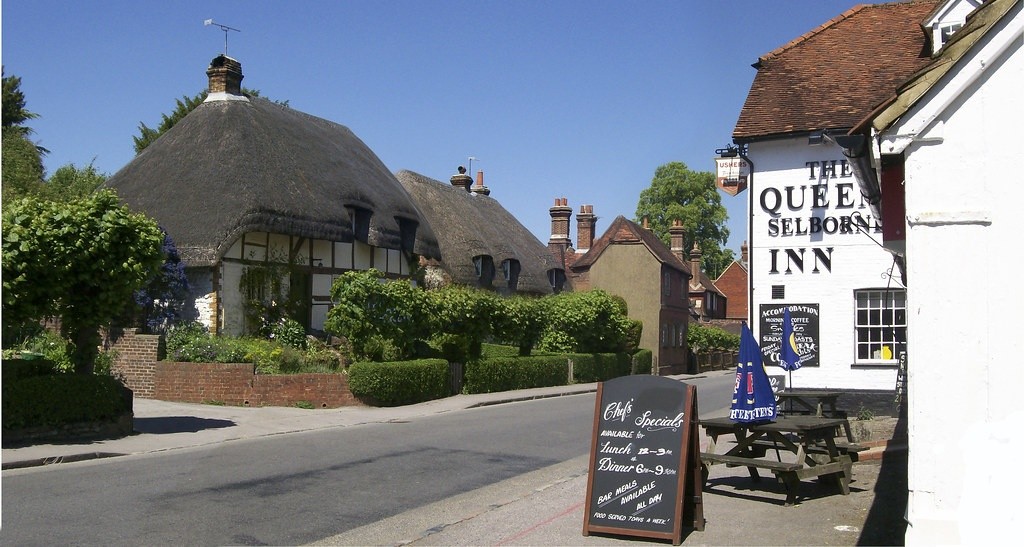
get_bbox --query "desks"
[774,391,851,437]
[700,416,870,506]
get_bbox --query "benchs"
[779,409,854,413]
[777,416,824,420]
[727,440,871,451]
[700,451,803,472]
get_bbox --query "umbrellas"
[780,308,802,416]
[728,321,782,462]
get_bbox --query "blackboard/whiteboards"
[583,375,706,547]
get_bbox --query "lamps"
[808,130,829,147]
